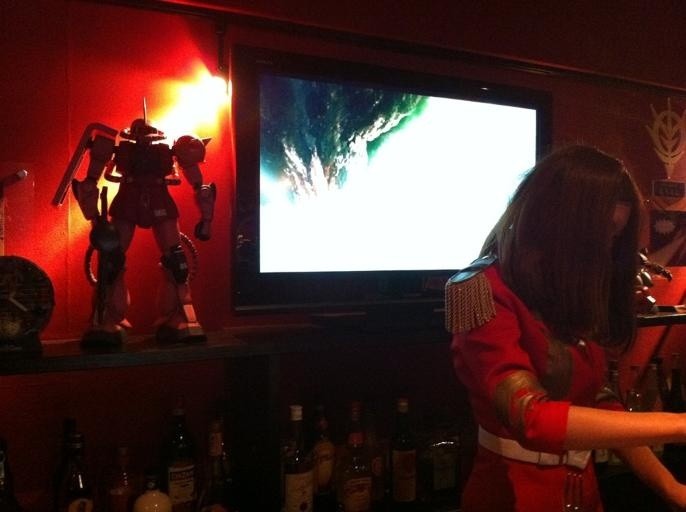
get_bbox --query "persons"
[443,140,686,511]
[70,114,218,351]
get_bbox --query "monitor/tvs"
[236,44,554,306]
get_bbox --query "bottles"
[53,357,686,512]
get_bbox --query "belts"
[478,423,568,466]
